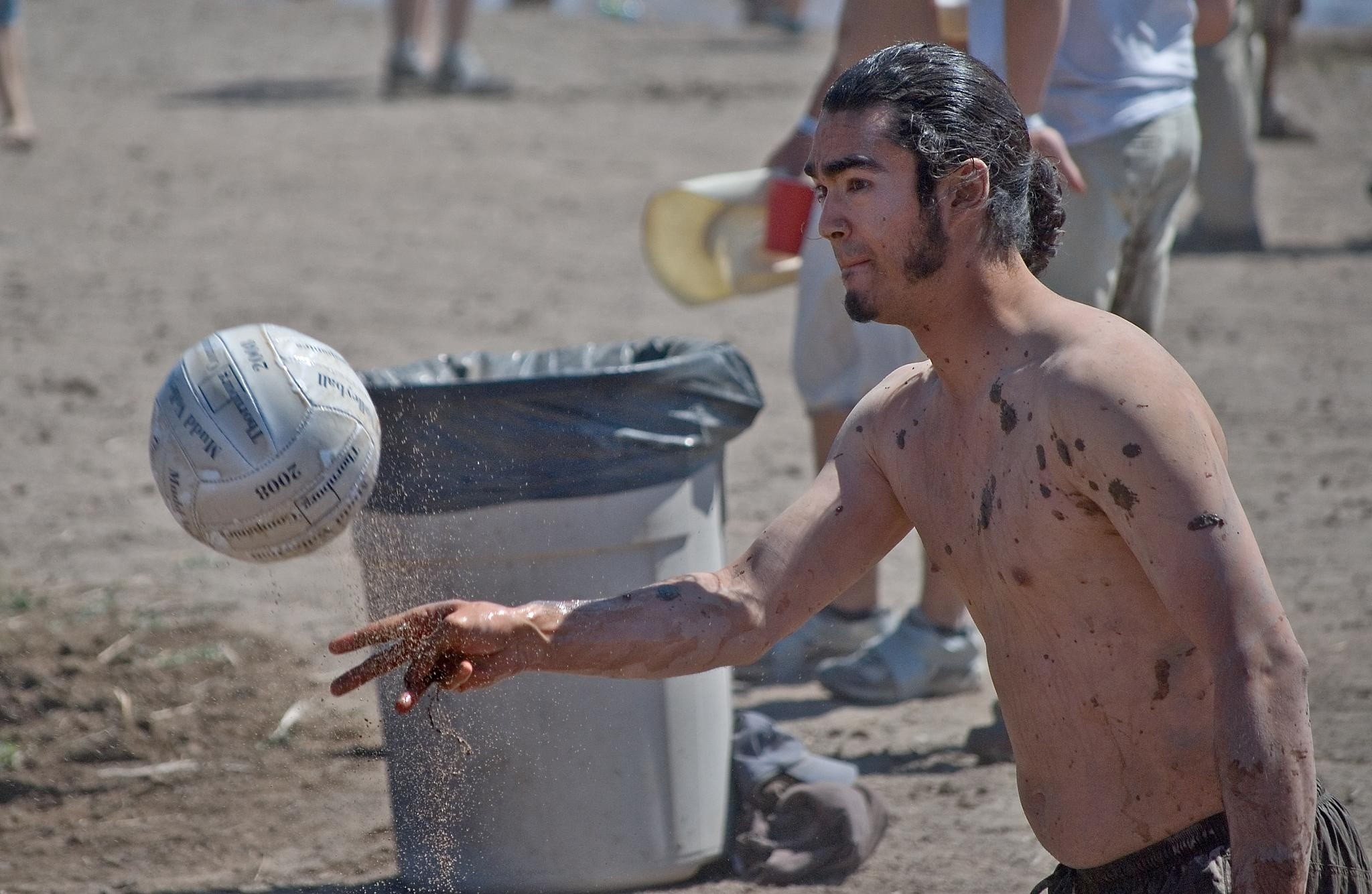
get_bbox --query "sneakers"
[734,606,897,688]
[814,604,982,704]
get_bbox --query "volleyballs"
[147,321,384,565]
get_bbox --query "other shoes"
[1259,111,1314,140]
[386,53,503,93]
[1176,216,1263,254]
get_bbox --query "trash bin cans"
[347,340,763,894]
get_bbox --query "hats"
[730,713,859,798]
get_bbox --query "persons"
[381,0,522,101]
[329,39,1372,894]
[732,0,1319,705]
[0,0,37,144]
